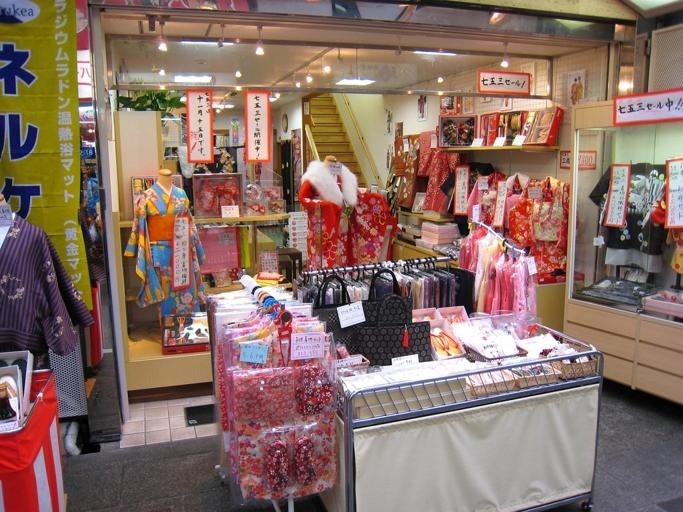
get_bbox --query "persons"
[570,78,577,105]
[297,154,358,271]
[576,76,584,100]
[0,192,95,366]
[124,169,206,331]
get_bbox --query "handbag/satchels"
[312,268,432,365]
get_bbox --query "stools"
[276,247,303,283]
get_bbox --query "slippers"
[295,367,334,416]
[266,441,288,492]
[294,435,316,485]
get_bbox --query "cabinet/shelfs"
[392,144,582,330]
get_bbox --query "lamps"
[153,20,265,56]
[500,42,511,70]
